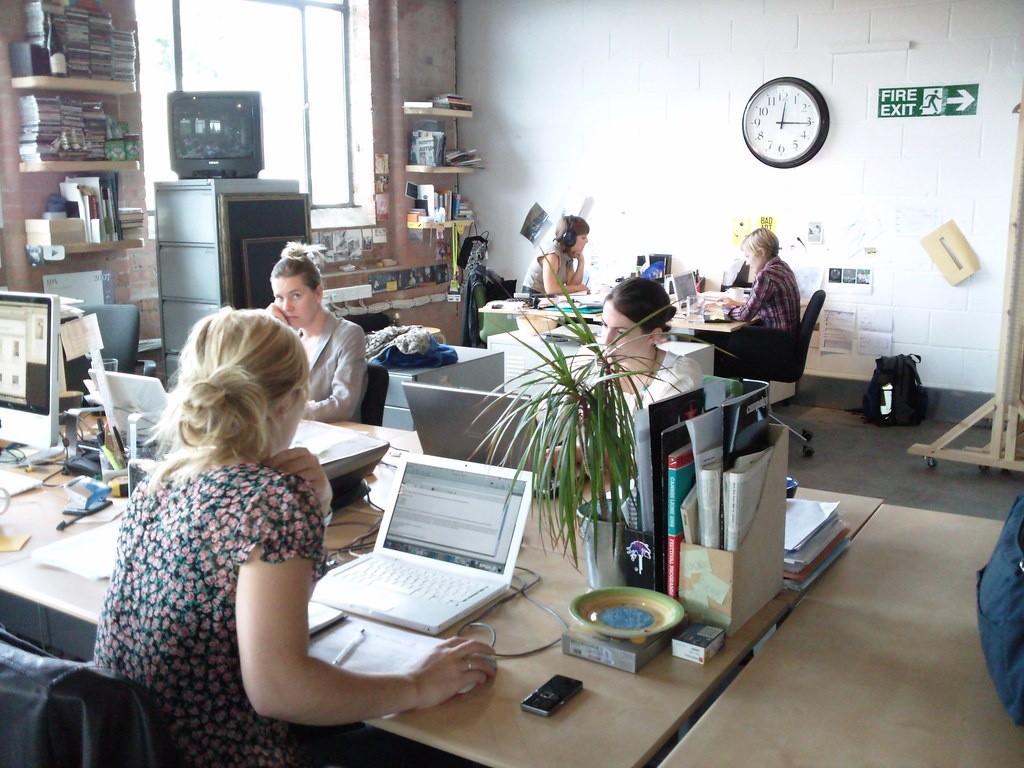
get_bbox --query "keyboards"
[0,469,43,499]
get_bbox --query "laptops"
[89,369,170,445]
[671,270,727,314]
[401,383,585,499]
[311,451,539,636]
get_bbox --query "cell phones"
[520,674,584,718]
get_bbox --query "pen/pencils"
[692,269,703,295]
[112,426,130,464]
[333,628,366,665]
[95,417,120,470]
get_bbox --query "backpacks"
[859,353,928,427]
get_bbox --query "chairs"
[690,289,826,469]
[460,264,518,348]
[77,303,157,377]
[0,621,190,768]
[361,363,390,425]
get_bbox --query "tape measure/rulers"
[107,475,128,497]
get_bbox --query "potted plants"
[465,242,743,590]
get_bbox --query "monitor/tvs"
[0,290,83,468]
[167,90,264,180]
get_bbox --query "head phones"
[563,216,577,247]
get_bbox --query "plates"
[568,586,685,636]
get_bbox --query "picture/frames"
[216,192,313,309]
[241,233,305,309]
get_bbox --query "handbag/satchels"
[457,222,489,270]
[973,494,1024,724]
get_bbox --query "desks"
[59,412,410,442]
[593,298,809,413]
[362,430,885,630]
[478,307,602,318]
[382,345,504,431]
[656,502,1024,768]
[0,467,790,768]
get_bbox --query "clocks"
[742,77,830,169]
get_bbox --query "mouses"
[456,654,496,693]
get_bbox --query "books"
[307,607,443,678]
[406,91,484,219]
[17,1,151,247]
[624,376,852,594]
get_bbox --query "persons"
[266,240,369,422]
[92,309,497,768]
[535,279,703,485]
[522,215,591,294]
[703,226,800,332]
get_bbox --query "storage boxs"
[24,218,86,246]
[671,622,726,665]
[561,612,690,674]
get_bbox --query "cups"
[91,359,119,372]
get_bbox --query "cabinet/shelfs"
[403,106,477,229]
[152,178,300,388]
[11,74,146,253]
